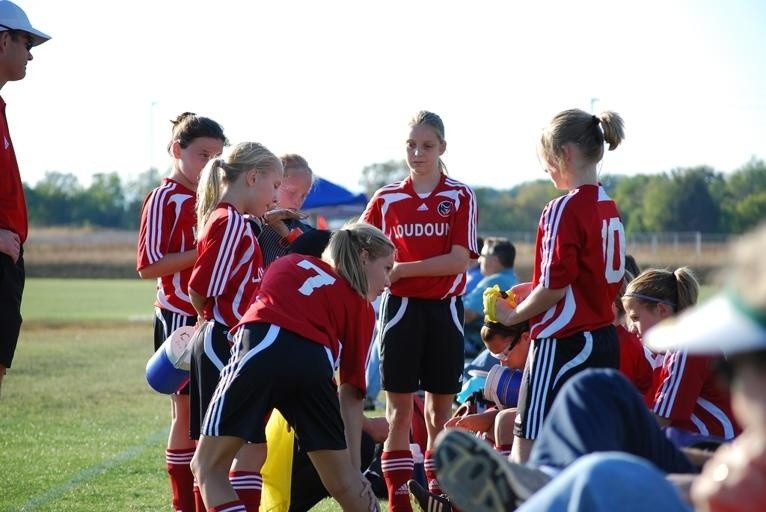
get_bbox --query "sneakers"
[407,479,454,512]
[431,429,552,512]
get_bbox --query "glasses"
[491,335,519,362]
[0,23,35,51]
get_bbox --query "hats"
[644,286,765,353]
[0,0,51,47]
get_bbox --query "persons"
[0,0,51,384]
[258,409,389,512]
[353,110,480,511]
[363,317,382,409]
[190,222,396,512]
[251,154,313,269]
[136,113,227,511]
[433,107,766,511]
[187,142,283,512]
